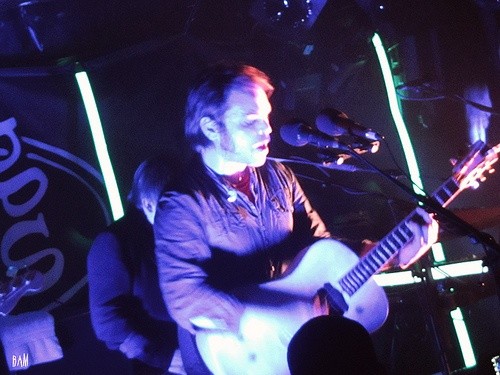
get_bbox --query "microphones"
[316,108,383,145]
[277,117,351,157]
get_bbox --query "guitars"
[176,140,500,375]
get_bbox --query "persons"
[85,154,192,375]
[151,59,442,374]
[455,58,493,148]
